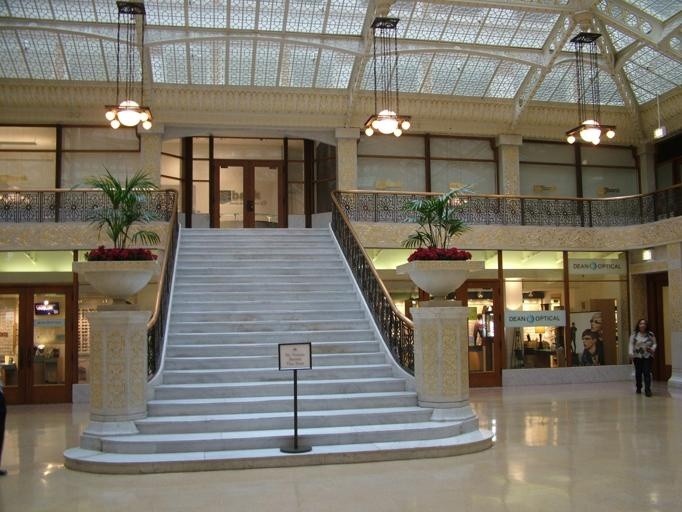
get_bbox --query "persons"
[33,346,61,384]
[570,322,577,353]
[0,390,9,475]
[473,313,484,346]
[546,329,557,367]
[628,319,657,397]
[0,355,18,385]
[580,329,605,366]
[590,312,603,342]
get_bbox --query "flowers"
[400,182,477,261]
[67,161,162,261]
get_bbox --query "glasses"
[582,338,593,340]
[589,319,601,324]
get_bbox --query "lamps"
[565,31,618,148]
[363,12,413,138]
[103,2,156,133]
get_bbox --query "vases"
[395,260,485,308]
[72,262,158,312]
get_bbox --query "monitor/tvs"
[35,303,59,315]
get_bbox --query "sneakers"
[636,388,651,397]
